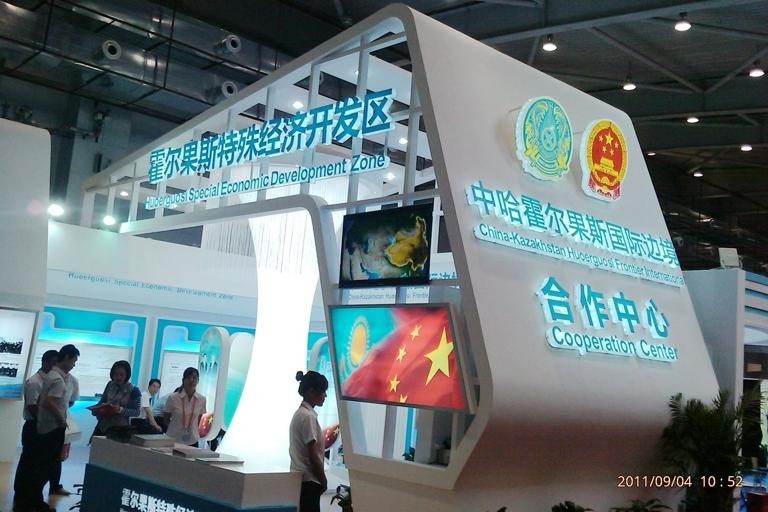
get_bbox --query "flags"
[340,301,466,408]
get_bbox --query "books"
[82,403,119,417]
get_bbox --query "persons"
[12,344,80,511]
[152,387,186,433]
[289,370,329,511]
[50,373,80,494]
[14,349,60,487]
[165,368,208,448]
[87,360,142,440]
[139,377,164,434]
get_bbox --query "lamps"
[749,59,765,77]
[675,12,691,32]
[623,62,637,90]
[542,34,557,52]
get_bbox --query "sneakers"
[49,485,71,496]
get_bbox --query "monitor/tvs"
[339,202,433,286]
[0,305,39,400]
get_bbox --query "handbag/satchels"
[106,425,139,443]
